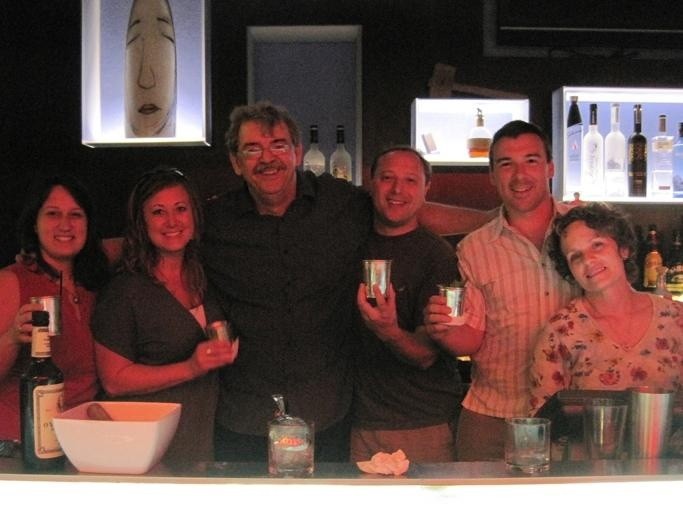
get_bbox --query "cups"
[29,295,61,339]
[206,319,237,347]
[362,259,391,299]
[585,397,627,460]
[266,418,316,476]
[626,385,675,460]
[438,283,466,320]
[506,416,554,473]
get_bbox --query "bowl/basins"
[52,400,185,477]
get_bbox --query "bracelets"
[204,348,211,354]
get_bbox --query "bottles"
[330,125,352,182]
[626,222,683,295]
[467,108,491,158]
[18,308,66,469]
[302,125,326,176]
[564,95,683,203]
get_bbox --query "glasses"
[235,144,291,156]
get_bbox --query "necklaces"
[39,270,81,304]
[582,290,637,353]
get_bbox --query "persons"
[419,120,573,460]
[0,177,100,461]
[12,101,569,465]
[340,142,464,467]
[123,1,177,137]
[526,198,682,456]
[87,160,239,468]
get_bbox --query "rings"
[14,325,22,335]
